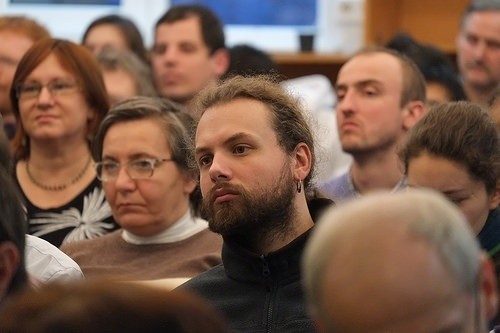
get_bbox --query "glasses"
[13,76,80,100]
[93,156,174,184]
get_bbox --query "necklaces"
[26,155,92,191]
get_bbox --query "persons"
[314,47,430,206]
[56,95,227,292]
[301,183,500,333]
[401,102,500,333]
[1,6,283,333]
[386,0,500,129]
[157,71,342,333]
